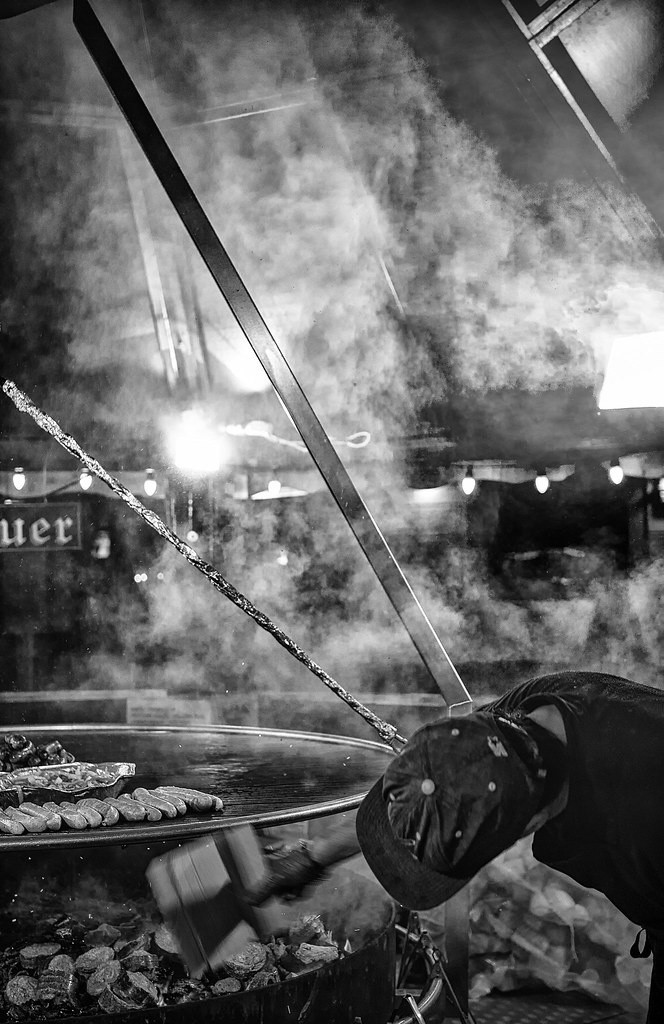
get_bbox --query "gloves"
[238,839,328,906]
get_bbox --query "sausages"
[0,731,67,772]
[0,786,223,836]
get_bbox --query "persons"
[247,668,664,1024]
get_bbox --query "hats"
[356,710,549,910]
